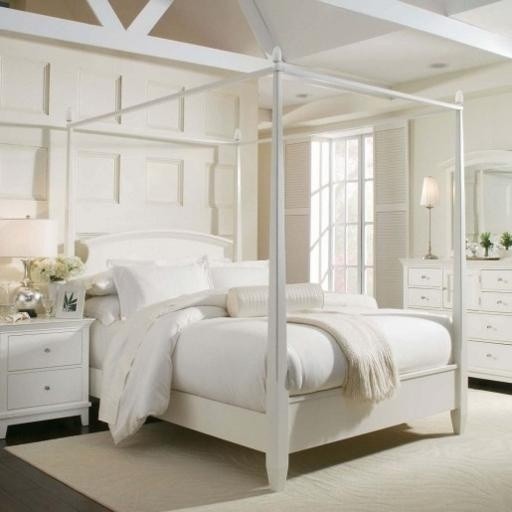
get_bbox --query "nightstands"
[0,317,98,440]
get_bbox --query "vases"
[46,277,65,311]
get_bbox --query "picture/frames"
[54,282,86,320]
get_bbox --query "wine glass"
[40,283,58,318]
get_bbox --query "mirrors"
[442,149,512,251]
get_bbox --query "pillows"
[83,294,120,326]
[82,270,117,297]
[106,257,212,319]
[226,283,326,320]
[205,259,269,291]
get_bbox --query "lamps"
[420,175,440,260]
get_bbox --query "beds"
[60,47,468,496]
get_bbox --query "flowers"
[30,255,84,281]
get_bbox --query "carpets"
[5,386,512,512]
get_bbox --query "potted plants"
[479,231,493,258]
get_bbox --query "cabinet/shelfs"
[395,255,512,387]
